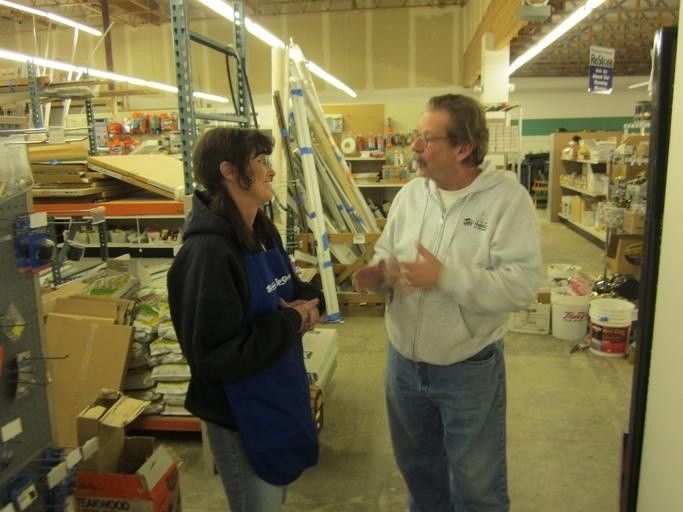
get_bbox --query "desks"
[519,161,549,198]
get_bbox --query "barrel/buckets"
[589,297,635,358]
[551,286,596,341]
[548,262,581,293]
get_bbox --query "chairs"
[532,178,550,211]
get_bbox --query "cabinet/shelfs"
[334,148,417,215]
[27,188,184,259]
[548,130,651,255]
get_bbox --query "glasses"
[411,129,453,146]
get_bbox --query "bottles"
[365,198,384,218]
[357,132,401,151]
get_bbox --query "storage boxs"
[74,432,182,512]
[621,208,646,233]
[609,238,643,276]
[508,289,552,335]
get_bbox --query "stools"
[603,255,611,277]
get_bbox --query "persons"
[352,93,543,511]
[165,124,321,511]
